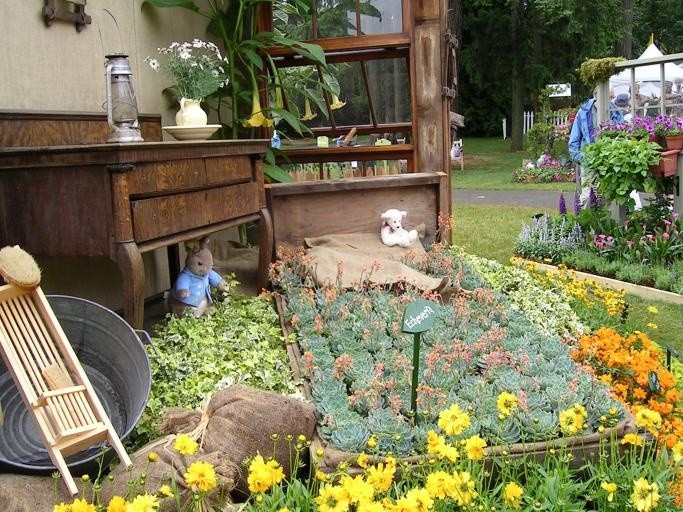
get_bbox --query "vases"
[161,98,222,142]
[664,135,683,151]
[646,135,662,146]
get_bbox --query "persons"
[627,84,651,121]
[614,94,632,118]
[654,80,682,115]
[567,85,622,210]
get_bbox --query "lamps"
[101,51,146,143]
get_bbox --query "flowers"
[591,109,683,138]
[142,36,230,99]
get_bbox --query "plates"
[160,122,223,140]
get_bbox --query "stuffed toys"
[166,237,228,320]
[379,209,418,247]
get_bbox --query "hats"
[614,92,629,106]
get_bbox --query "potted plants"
[579,138,680,206]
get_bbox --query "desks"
[0,138,273,342]
